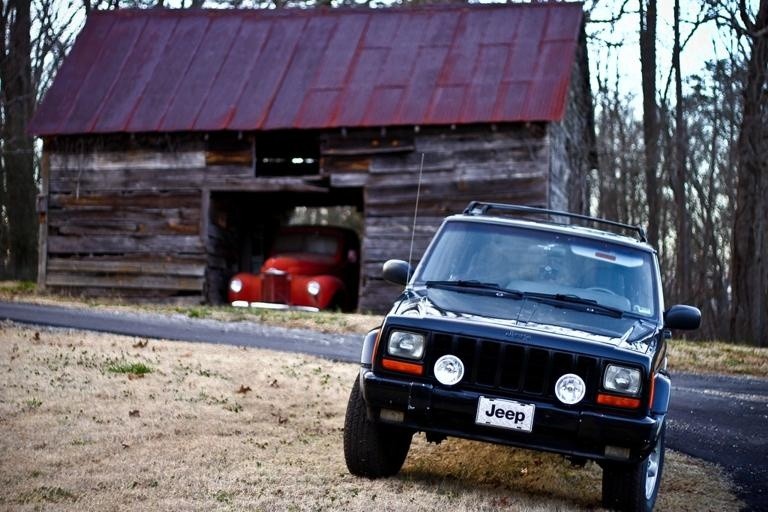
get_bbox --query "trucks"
[228,225,360,312]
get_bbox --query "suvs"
[343,198,702,511]
